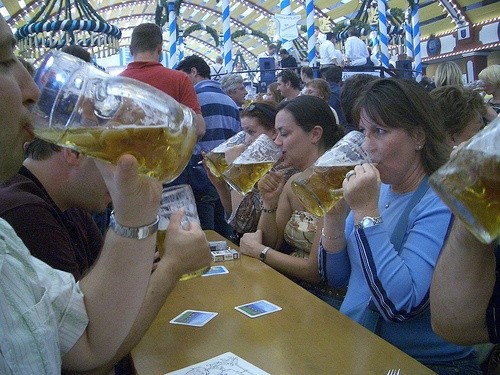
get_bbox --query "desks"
[129,229,435,374]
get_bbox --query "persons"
[257,26,385,136]
[430,141,500,375]
[239,95,344,291]
[116,22,206,189]
[197,100,280,246]
[428,60,500,146]
[209,55,243,81]
[0,13,162,375]
[1,137,212,375]
[62,45,91,65]
[175,54,243,240]
[317,77,482,375]
[218,74,248,108]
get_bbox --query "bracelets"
[321,227,346,240]
[261,204,278,213]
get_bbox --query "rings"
[345,170,356,181]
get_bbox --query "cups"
[242,97,253,109]
[205,131,255,177]
[290,131,380,216]
[154,184,212,281]
[468,79,486,92]
[427,113,500,242]
[221,134,284,195]
[25,50,199,184]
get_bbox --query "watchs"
[354,216,383,230]
[259,246,270,262]
[110,209,159,240]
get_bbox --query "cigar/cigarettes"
[197,160,203,165]
